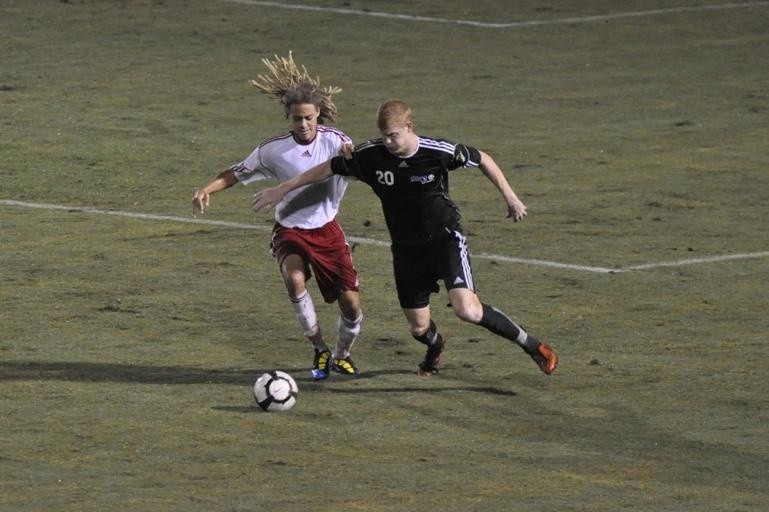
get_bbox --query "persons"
[191,50,363,382]
[248,101,558,376]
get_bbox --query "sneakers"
[417,334,447,377]
[329,354,361,378]
[310,345,333,381]
[521,331,560,376]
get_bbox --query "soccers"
[254,371,298,411]
